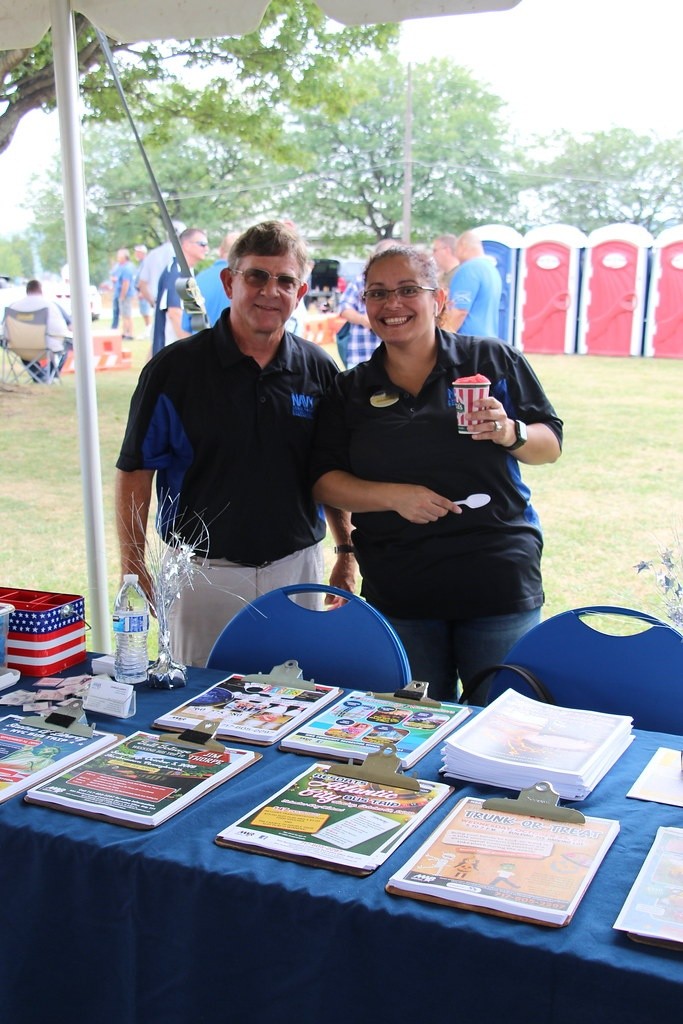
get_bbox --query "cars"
[46,280,101,319]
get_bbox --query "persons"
[0,219,245,384]
[117,223,358,668]
[303,246,563,707]
[0,737,61,772]
[306,230,502,373]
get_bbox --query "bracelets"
[150,300,155,308]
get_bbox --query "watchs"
[335,545,354,554]
[501,420,528,451]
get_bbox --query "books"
[439,688,636,803]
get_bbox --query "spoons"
[454,493,491,509]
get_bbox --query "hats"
[135,245,147,254]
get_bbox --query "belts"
[168,546,302,569]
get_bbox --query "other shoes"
[122,334,133,341]
[136,331,151,340]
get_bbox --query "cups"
[452,382,490,434]
[0,602,15,677]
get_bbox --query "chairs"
[484,606,683,735]
[204,584,412,693]
[0,307,66,385]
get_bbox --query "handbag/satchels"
[336,322,350,368]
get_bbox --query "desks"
[0,652,683,1024]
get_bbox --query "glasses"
[363,285,435,300]
[190,241,207,247]
[228,267,302,294]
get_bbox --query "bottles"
[112,574,150,684]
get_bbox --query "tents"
[0,0,523,655]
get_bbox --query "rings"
[494,421,502,432]
